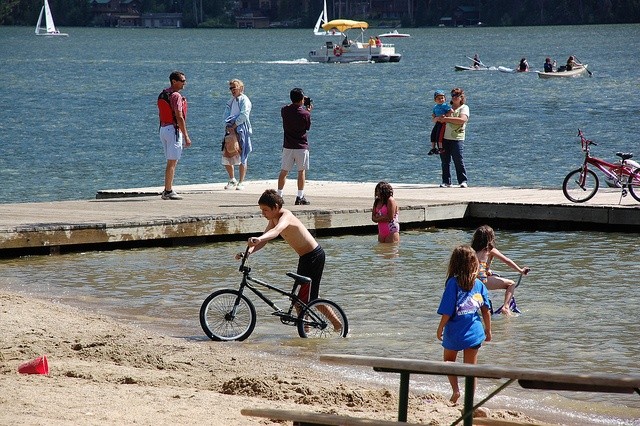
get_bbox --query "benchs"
[321,353,640,426]
[240,407,430,426]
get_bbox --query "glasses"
[177,78,186,82]
[451,93,460,97]
[229,86,235,90]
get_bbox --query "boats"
[455,65,498,71]
[378,32,411,38]
[499,65,540,73]
[538,63,588,78]
[307,18,402,63]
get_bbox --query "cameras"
[304,97,313,106]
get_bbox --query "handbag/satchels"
[221,125,242,150]
[224,128,242,157]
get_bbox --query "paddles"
[464,55,496,70]
[572,55,593,76]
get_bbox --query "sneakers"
[161,189,182,199]
[295,195,310,205]
[440,183,453,187]
[162,191,183,200]
[427,147,437,155]
[236,180,246,190]
[435,148,445,153]
[225,179,238,189]
[460,180,467,187]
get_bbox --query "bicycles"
[488,269,532,315]
[199,240,348,341]
[562,128,640,205]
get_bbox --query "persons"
[471,225,530,316]
[374,36,380,46]
[519,57,528,71]
[236,190,343,334]
[427,89,454,156]
[342,36,350,48]
[440,87,469,187]
[223,78,252,191]
[277,88,313,205]
[369,37,375,48]
[473,53,481,70]
[437,245,492,417]
[158,71,190,200]
[567,55,581,71]
[544,58,557,73]
[372,182,400,243]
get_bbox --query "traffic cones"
[17,354,50,376]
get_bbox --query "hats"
[434,89,445,98]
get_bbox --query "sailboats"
[313,0,343,35]
[34,0,69,36]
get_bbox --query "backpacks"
[168,90,187,143]
